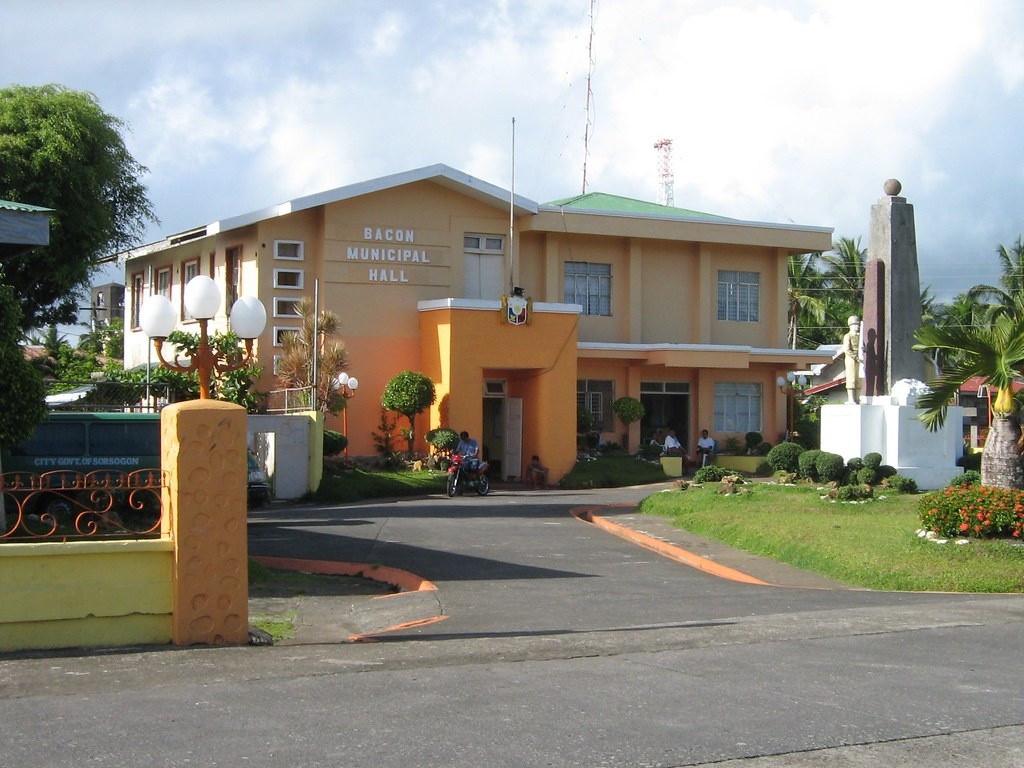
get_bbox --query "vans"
[0,413,276,533]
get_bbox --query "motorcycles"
[445,452,489,498]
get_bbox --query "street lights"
[139,274,267,400]
[331,372,359,466]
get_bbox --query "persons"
[526,456,550,490]
[843,316,863,404]
[449,431,488,480]
[665,428,692,476]
[650,432,665,456]
[696,429,715,467]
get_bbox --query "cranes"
[654,138,674,210]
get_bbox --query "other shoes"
[544,486,550,489]
[534,486,540,490]
[469,481,475,487]
